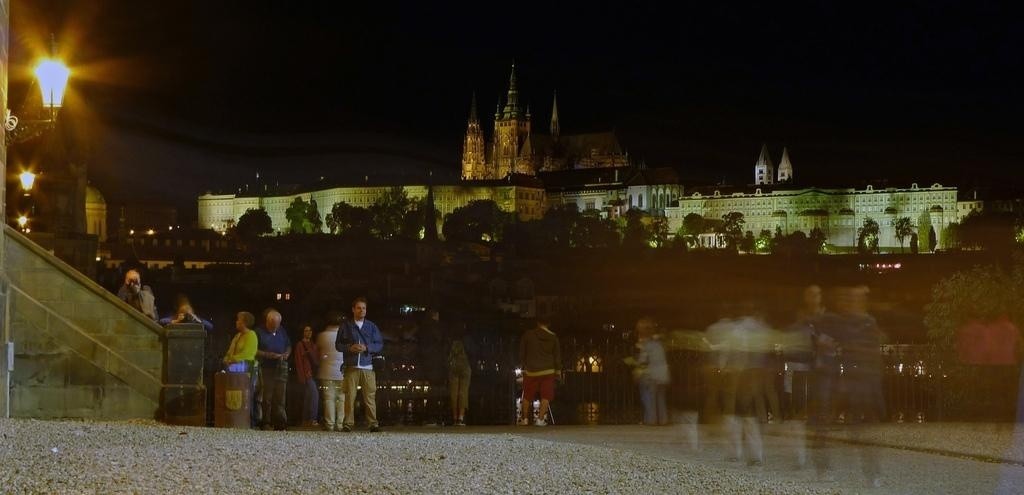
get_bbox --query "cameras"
[128,279,139,288]
[184,313,194,323]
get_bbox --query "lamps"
[6,32,75,142]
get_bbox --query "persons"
[516,315,562,427]
[621,319,669,425]
[445,316,475,425]
[117,266,384,431]
[954,288,1023,442]
[668,277,886,487]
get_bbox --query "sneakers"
[452,419,466,426]
[519,418,529,426]
[536,418,548,426]
